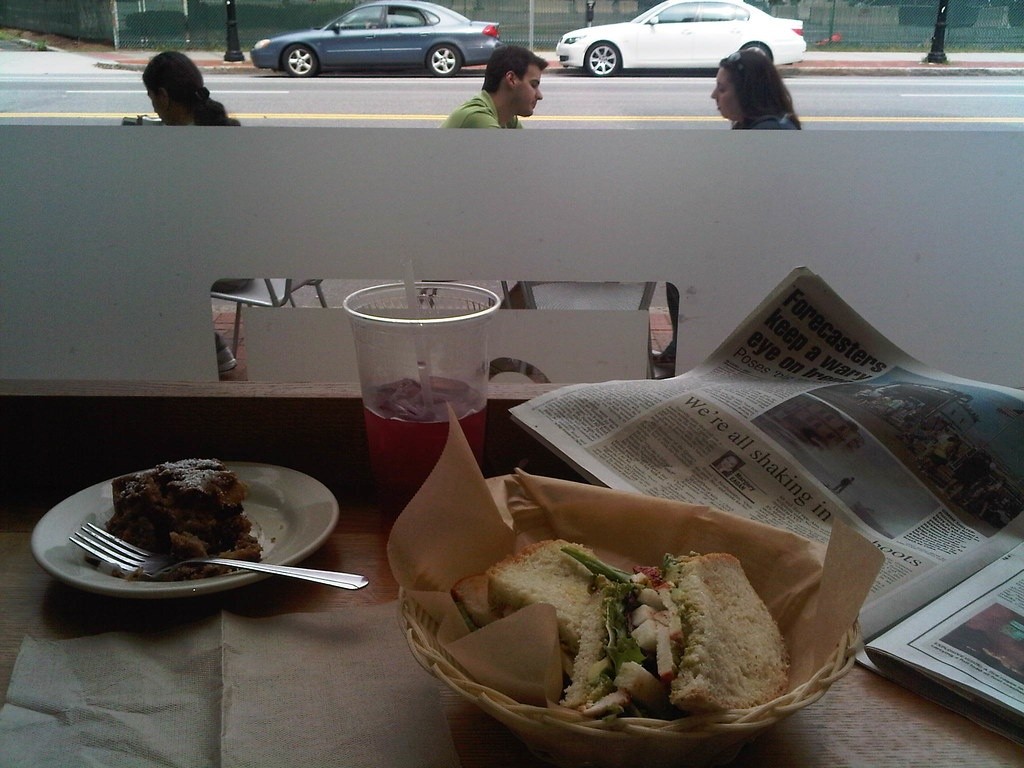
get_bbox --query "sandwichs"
[484,539,595,685]
[451,574,489,631]
[562,554,787,715]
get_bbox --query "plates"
[30,461,338,597]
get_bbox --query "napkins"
[0,600,462,768]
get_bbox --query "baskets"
[397,505,863,768]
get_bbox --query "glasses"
[727,51,745,71]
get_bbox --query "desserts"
[103,457,261,580]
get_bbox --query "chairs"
[210,277,327,358]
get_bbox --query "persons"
[860,389,912,416]
[834,476,855,494]
[142,50,253,372]
[653,51,801,370]
[433,45,550,129]
[930,435,1006,516]
[713,454,741,478]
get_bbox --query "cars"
[250,0,500,77]
[556,0,808,77]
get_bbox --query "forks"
[69,523,368,589]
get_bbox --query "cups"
[343,281,501,497]
